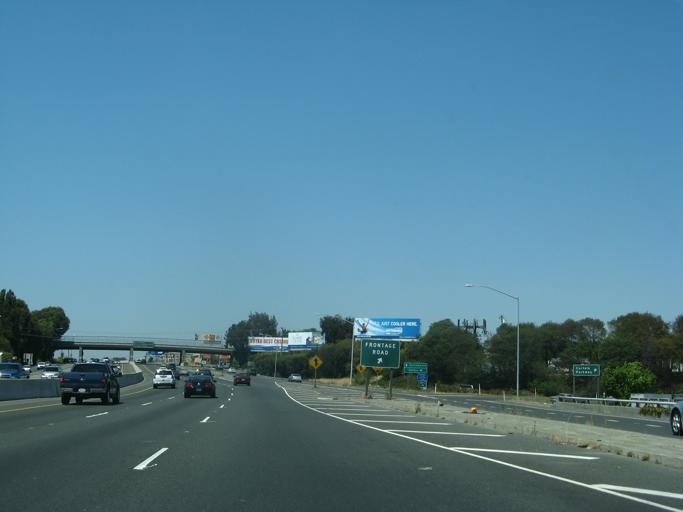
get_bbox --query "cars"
[668,401,683,438]
[40,365,63,379]
[246,367,257,376]
[288,374,303,383]
[37,361,46,369]
[226,367,236,373]
[44,360,52,366]
[62,357,78,363]
[85,356,124,377]
[23,365,31,373]
[232,372,250,386]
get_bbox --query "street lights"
[313,311,355,387]
[462,283,523,394]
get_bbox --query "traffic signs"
[573,364,600,376]
[403,363,428,374]
[360,338,401,368]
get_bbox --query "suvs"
[151,361,218,399]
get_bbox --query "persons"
[358,319,370,334]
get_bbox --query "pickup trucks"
[0,360,31,379]
[58,363,121,402]
